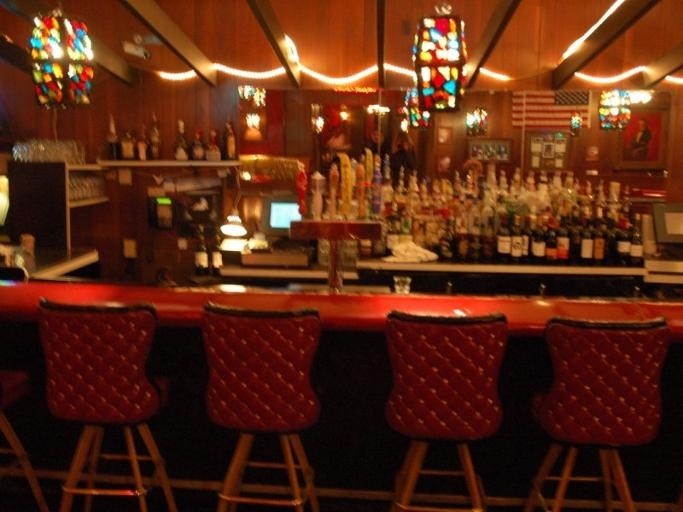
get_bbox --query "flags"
[512,89,591,134]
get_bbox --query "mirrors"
[230,87,674,201]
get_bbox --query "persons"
[630,118,651,161]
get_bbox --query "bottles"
[193,228,209,276]
[105,112,236,160]
[211,233,222,273]
[307,148,657,269]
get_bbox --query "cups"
[391,276,411,295]
[67,173,103,202]
[19,231,36,254]
[14,138,86,165]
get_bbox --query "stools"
[0,370,51,510]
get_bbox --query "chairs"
[385,310,509,512]
[201,300,322,512]
[37,295,180,512]
[522,315,671,512]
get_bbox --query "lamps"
[401,88,429,129]
[405,0,463,112]
[26,1,91,109]
[568,105,582,132]
[598,89,630,131]
[466,106,488,136]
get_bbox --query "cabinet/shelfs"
[5,157,110,254]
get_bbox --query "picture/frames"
[529,133,567,169]
[436,126,452,145]
[436,153,454,178]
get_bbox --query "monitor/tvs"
[261,195,303,236]
